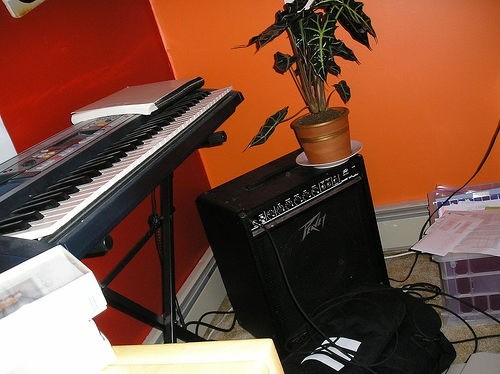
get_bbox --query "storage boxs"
[428,183,500,327]
[0,244,116,374]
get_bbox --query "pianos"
[0,76,245,264]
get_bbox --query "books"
[71,76,205,125]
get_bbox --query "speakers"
[196,147,391,340]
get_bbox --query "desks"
[113,338,285,374]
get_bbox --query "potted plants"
[231,0,378,165]
